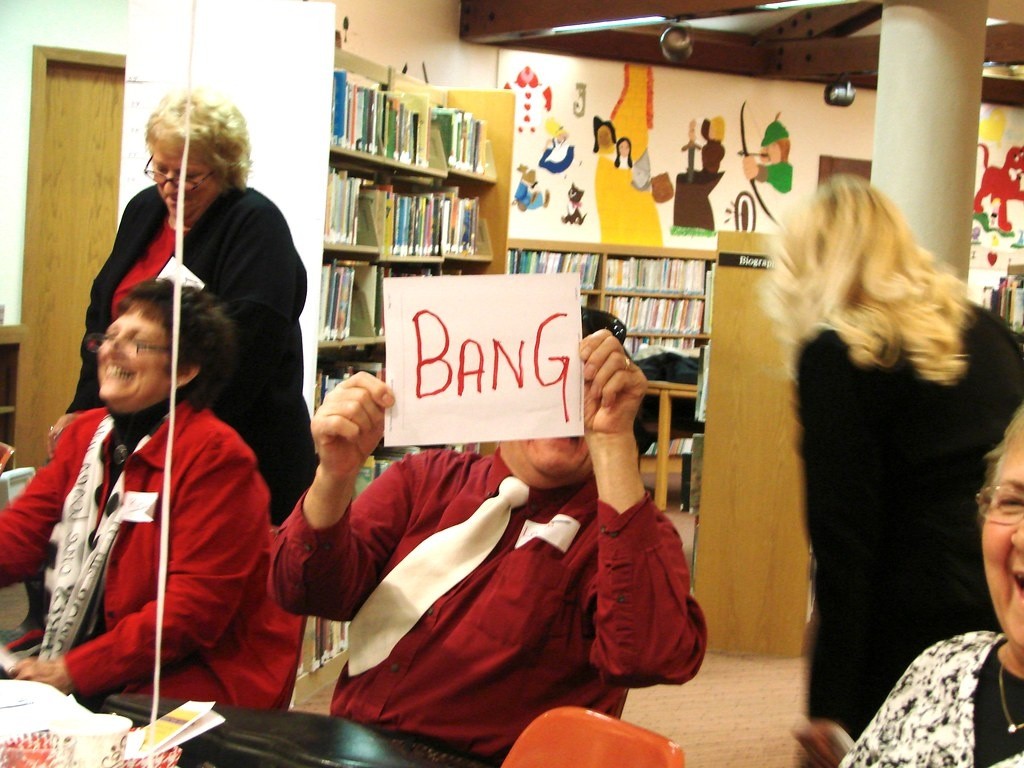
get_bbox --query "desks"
[642,379,698,513]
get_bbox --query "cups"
[53,715,133,768]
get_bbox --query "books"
[606,256,705,295]
[644,433,705,515]
[622,337,695,356]
[327,169,480,255]
[330,70,488,175]
[508,250,601,290]
[376,269,432,335]
[580,293,704,334]
[695,262,716,422]
[982,277,1024,332]
[321,263,357,343]
[317,357,480,501]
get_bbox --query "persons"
[766,173,1024,768]
[0,279,308,715]
[266,308,709,768]
[48,96,318,526]
[832,408,1024,768]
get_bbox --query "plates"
[3,730,182,768]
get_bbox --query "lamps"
[656,17,696,66]
[823,72,856,106]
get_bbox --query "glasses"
[81,332,176,362]
[143,154,215,195]
[974,486,1024,524]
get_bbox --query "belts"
[385,736,483,768]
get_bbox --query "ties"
[347,476,529,677]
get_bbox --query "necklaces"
[999,664,1024,733]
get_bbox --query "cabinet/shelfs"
[303,45,515,677]
[505,239,719,476]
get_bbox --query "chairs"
[501,704,688,768]
